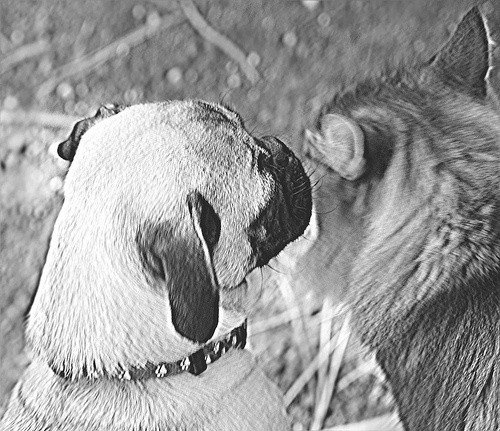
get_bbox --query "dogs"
[300,5,500,431]
[0,98,314,431]
